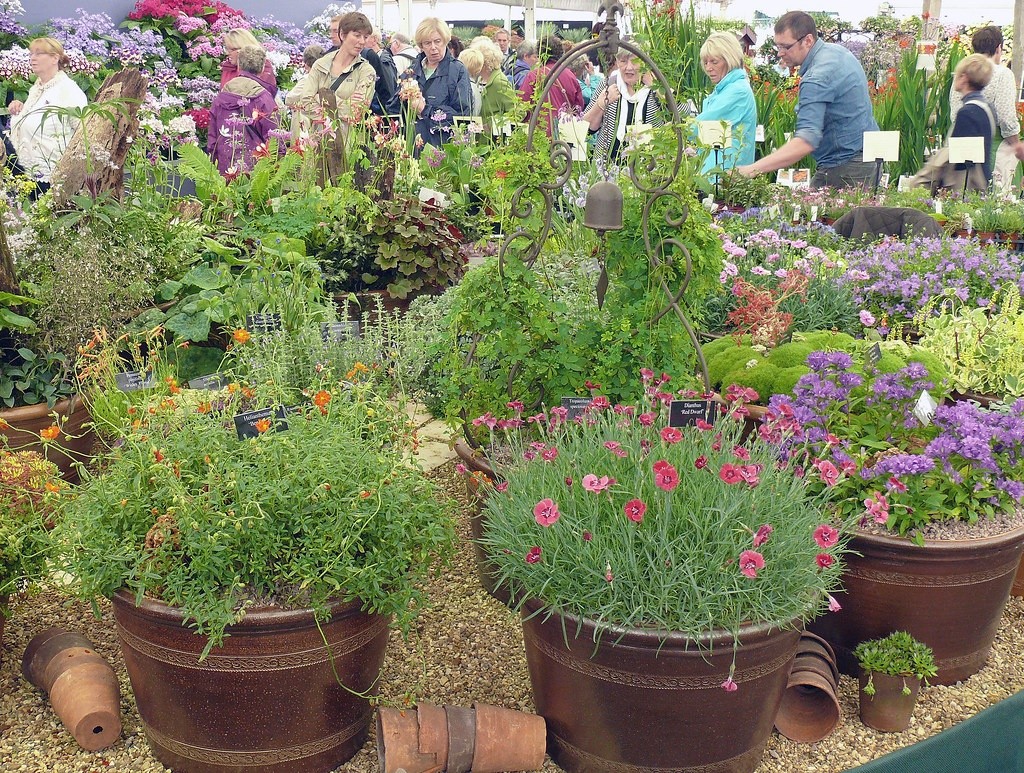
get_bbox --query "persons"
[578,39,691,168]
[952,53,995,192]
[7,37,88,198]
[206,8,608,186]
[944,25,1024,174]
[721,11,883,191]
[683,31,757,184]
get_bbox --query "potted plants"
[852,631,939,733]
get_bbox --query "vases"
[714,203,1018,253]
[0,287,1024,773]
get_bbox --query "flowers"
[459,364,864,643]
[12,286,461,703]
[759,344,1024,533]
[707,164,1024,233]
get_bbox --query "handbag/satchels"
[910,100,997,194]
[386,86,408,115]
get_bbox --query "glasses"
[28,50,50,59]
[772,33,809,53]
[387,40,396,48]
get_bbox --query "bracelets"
[596,100,608,111]
